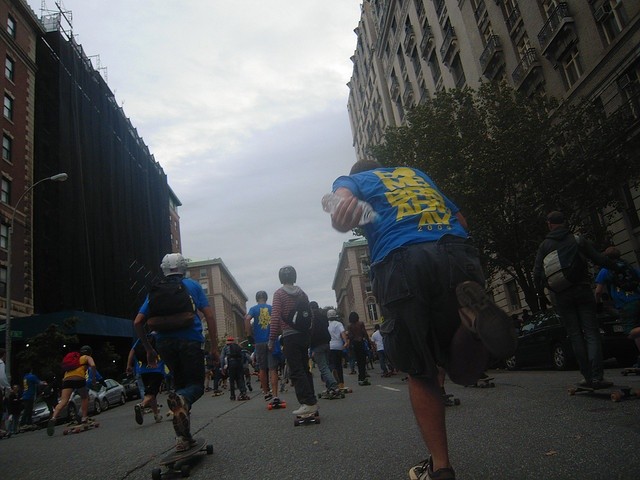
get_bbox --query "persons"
[220,335,247,400]
[245,291,281,402]
[533,211,615,389]
[327,309,348,389]
[347,312,374,386]
[66,365,107,424]
[0,348,12,439]
[371,324,394,377]
[20,365,48,429]
[134,252,217,452]
[203,349,227,394]
[241,346,254,392]
[309,301,341,395]
[126,330,170,425]
[321,160,519,480]
[47,345,96,436]
[268,267,320,415]
[595,247,640,367]
[5,384,23,437]
[44,384,59,416]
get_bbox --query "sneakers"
[273,397,279,403]
[175,435,199,451]
[134,403,144,425]
[265,394,274,401]
[82,416,93,424]
[247,383,252,391]
[292,404,317,415]
[153,414,163,423]
[409,454,455,480]
[47,419,57,436]
[456,280,518,359]
[166,392,190,437]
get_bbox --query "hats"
[545,211,565,224]
[600,246,621,260]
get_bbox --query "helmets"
[279,266,296,284]
[160,252,187,277]
[79,345,92,356]
[326,309,338,319]
[256,291,268,302]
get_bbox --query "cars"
[66,389,102,419]
[32,390,75,426]
[124,377,141,399]
[99,379,126,412]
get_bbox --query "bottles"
[322,193,381,226]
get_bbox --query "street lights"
[5,172,69,387]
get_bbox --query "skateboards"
[152,436,213,480]
[620,367,639,376]
[237,393,250,400]
[566,378,632,402]
[317,387,347,400]
[64,419,100,434]
[339,384,353,392]
[380,373,391,377]
[294,410,320,425]
[464,376,496,388]
[212,391,224,396]
[442,393,460,406]
[142,403,163,413]
[19,424,40,433]
[266,399,285,410]
[359,379,371,386]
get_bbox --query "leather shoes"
[592,381,614,390]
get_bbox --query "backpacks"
[230,343,242,357]
[600,258,640,292]
[280,291,313,330]
[60,352,88,372]
[541,234,586,295]
[144,276,194,331]
